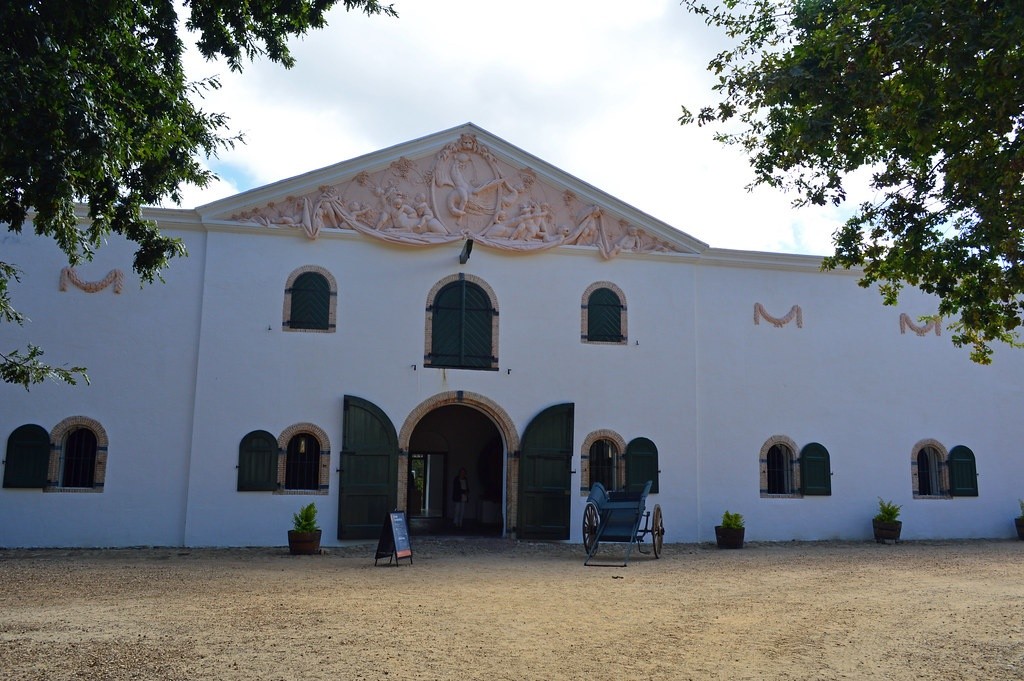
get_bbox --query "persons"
[232,185,447,235]
[453,469,470,528]
[485,202,670,251]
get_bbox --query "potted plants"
[873,497,904,544]
[1014,497,1024,542]
[715,512,746,550]
[287,503,323,555]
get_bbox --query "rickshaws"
[582,478,666,568]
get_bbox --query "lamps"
[459,238,475,264]
